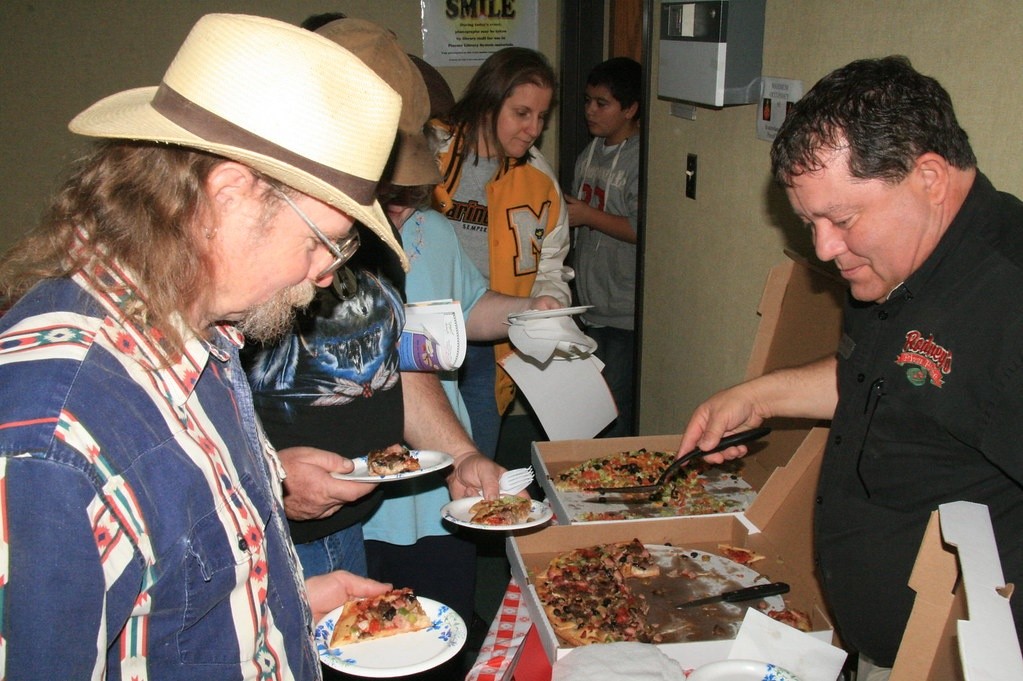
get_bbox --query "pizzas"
[716,544,766,564]
[767,609,812,633]
[468,495,532,526]
[555,448,726,522]
[329,587,433,647]
[366,444,421,476]
[534,538,661,649]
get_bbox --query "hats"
[309,19,447,185]
[65,14,412,284]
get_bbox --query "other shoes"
[465,610,489,652]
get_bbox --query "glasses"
[247,171,361,280]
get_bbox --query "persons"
[300,12,348,32]
[238,17,532,580]
[0,12,410,681]
[425,47,570,461]
[566,57,642,438]
[362,53,564,681]
[676,53,1023,681]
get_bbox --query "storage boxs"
[504,249,1023,681]
